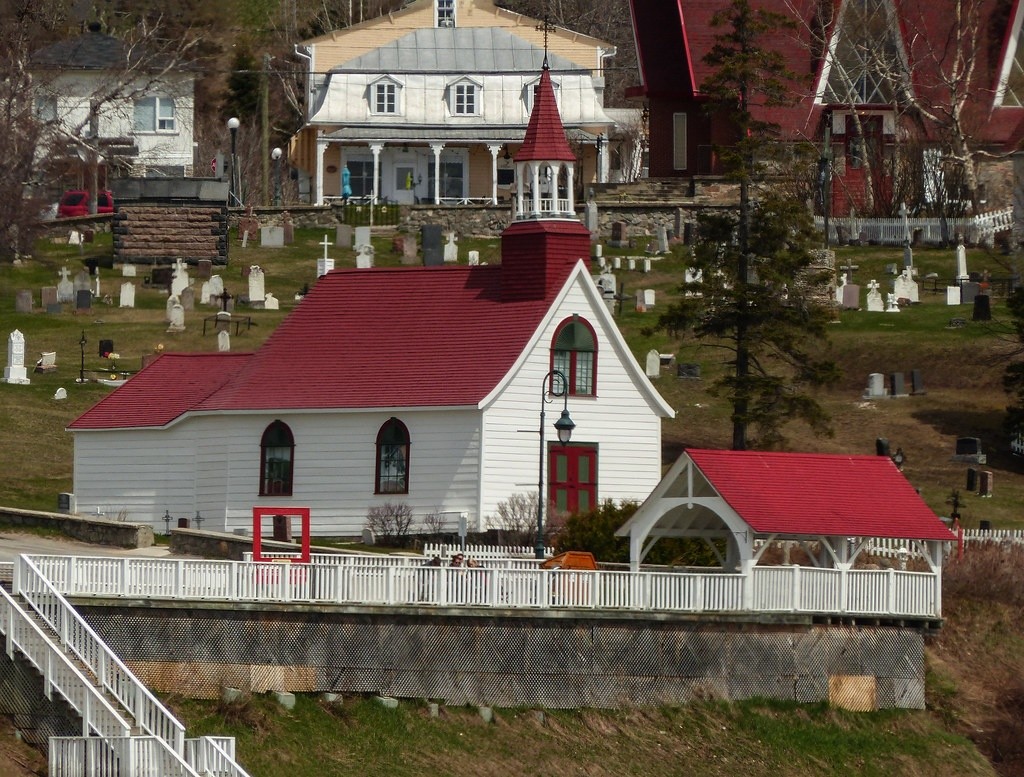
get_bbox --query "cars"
[55,190,113,219]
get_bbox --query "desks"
[79,370,140,380]
[204,315,251,337]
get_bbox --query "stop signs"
[211,158,216,174]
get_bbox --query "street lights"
[227,118,241,205]
[536,371,574,554]
[271,147,282,204]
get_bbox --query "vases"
[111,360,117,371]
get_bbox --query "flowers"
[104,352,120,360]
